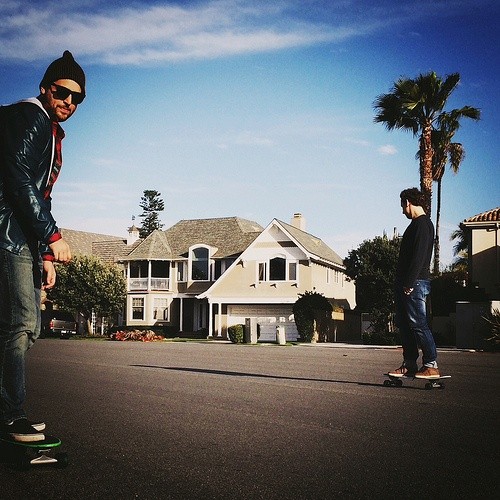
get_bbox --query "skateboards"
[383,372,452,390]
[0,433,70,472]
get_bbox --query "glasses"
[49,81,84,104]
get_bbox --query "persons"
[386,188,440,378]
[0,57,86,441]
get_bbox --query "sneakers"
[388,365,418,379]
[414,364,440,378]
[31,421,45,431]
[0,418,45,441]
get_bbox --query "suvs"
[40,309,77,340]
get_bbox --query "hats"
[40,51,85,97]
